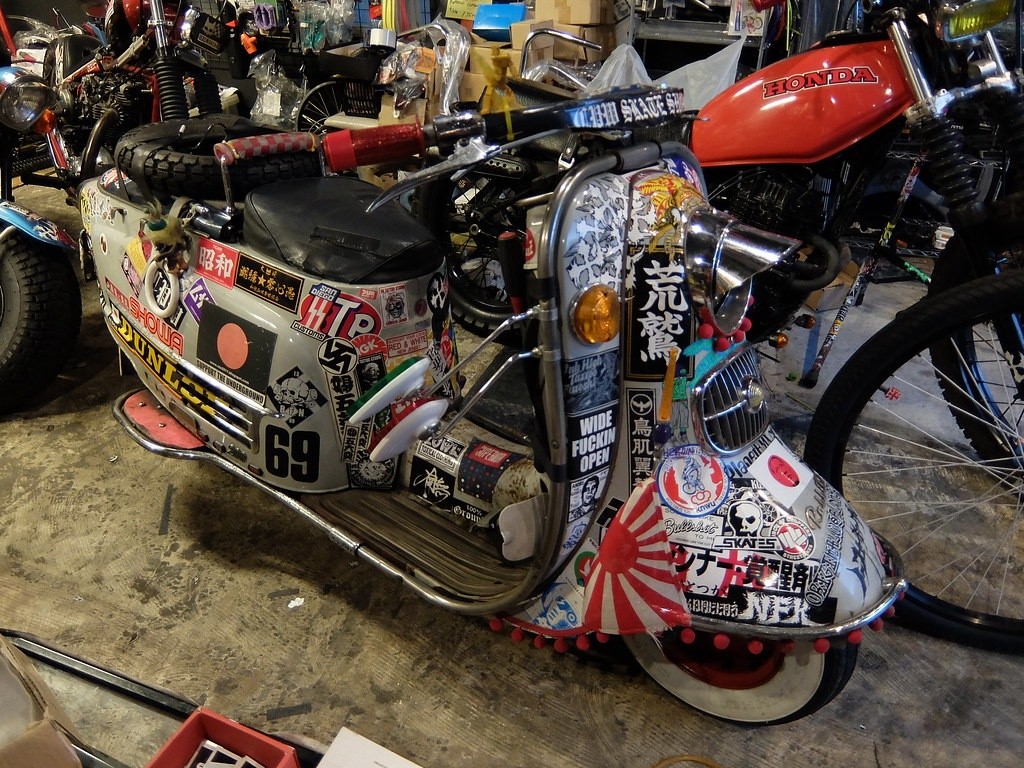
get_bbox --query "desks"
[325,111,379,130]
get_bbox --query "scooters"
[77,73,908,727]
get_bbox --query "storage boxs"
[797,245,858,314]
[188,94,239,117]
[334,74,383,119]
[360,0,615,192]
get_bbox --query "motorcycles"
[0,0,390,412]
[435,1,1024,658]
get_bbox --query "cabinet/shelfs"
[839,149,1003,257]
